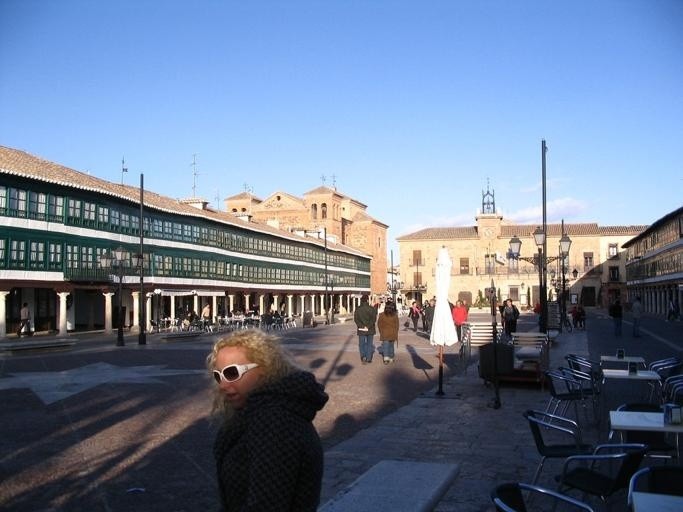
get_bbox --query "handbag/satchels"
[404,322,409,327]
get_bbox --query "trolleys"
[475,342,549,389]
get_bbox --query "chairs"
[489,352,682,512]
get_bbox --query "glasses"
[212,362,259,385]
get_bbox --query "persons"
[612,299,623,337]
[183,304,286,330]
[571,304,585,329]
[448,299,469,343]
[205,328,329,511]
[665,296,678,322]
[534,297,540,325]
[378,300,399,364]
[624,302,633,312]
[17,301,31,338]
[408,295,436,334]
[499,298,519,341]
[356,294,376,365]
[404,295,408,310]
[373,294,402,316]
[631,296,643,337]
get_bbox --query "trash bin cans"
[303,311,313,328]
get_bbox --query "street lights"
[386,280,404,308]
[99,244,144,347]
[414,282,427,303]
[318,274,344,326]
[549,266,578,318]
[505,225,575,332]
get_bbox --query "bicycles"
[558,315,573,334]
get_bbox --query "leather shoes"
[362,356,394,365]
